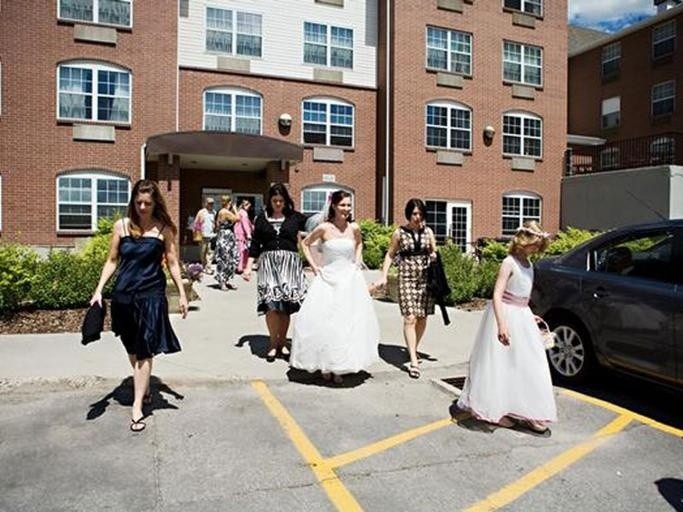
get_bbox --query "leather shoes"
[520,417,549,433]
[279,344,290,358]
[266,347,277,362]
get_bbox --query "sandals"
[410,364,420,378]
[498,416,516,428]
[130,408,146,431]
[143,391,153,407]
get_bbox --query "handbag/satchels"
[535,319,557,350]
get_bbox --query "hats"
[207,197,215,203]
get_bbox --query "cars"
[530,187,683,406]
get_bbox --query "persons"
[234,200,255,274]
[191,198,215,277]
[299,191,374,385]
[373,199,438,378]
[243,182,316,364]
[88,179,188,433]
[214,195,239,292]
[456,221,551,434]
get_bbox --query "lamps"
[278,113,292,133]
[484,125,494,146]
